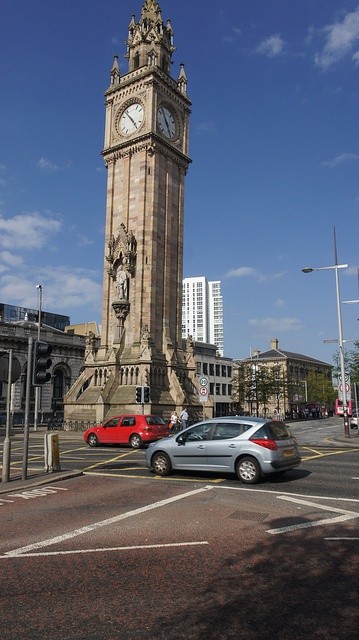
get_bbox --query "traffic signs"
[198,378,209,402]
[337,372,350,400]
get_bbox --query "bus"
[334,399,353,417]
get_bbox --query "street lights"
[301,224,359,440]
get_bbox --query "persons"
[180,406,190,430]
[168,411,179,434]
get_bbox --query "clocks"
[157,97,183,144]
[112,95,146,138]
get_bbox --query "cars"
[350,413,359,429]
[83,413,170,448]
[144,416,301,484]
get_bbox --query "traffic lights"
[135,386,142,403]
[143,386,150,404]
[34,339,52,386]
